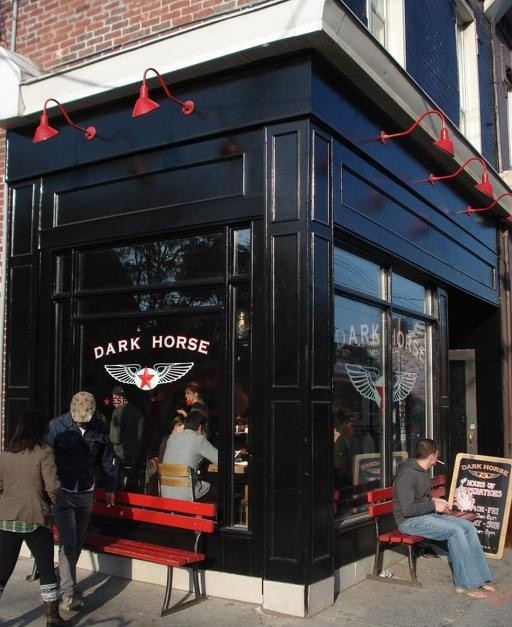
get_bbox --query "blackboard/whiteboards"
[448,452,512,560]
[352,452,409,513]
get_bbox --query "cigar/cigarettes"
[436,459,446,466]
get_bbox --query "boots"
[44,599,72,627]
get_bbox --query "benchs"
[337,479,382,519]
[25,489,222,618]
[367,476,477,586]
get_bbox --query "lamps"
[378,106,456,159]
[461,192,511,223]
[32,97,99,147]
[128,67,196,118]
[427,155,494,198]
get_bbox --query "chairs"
[154,460,223,527]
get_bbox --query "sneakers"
[62,597,84,612]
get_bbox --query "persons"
[333,401,383,488]
[98,380,219,501]
[47,387,122,614]
[391,437,496,600]
[1,401,73,625]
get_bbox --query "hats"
[69,391,96,423]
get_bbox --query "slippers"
[456,587,488,598]
[480,581,496,592]
